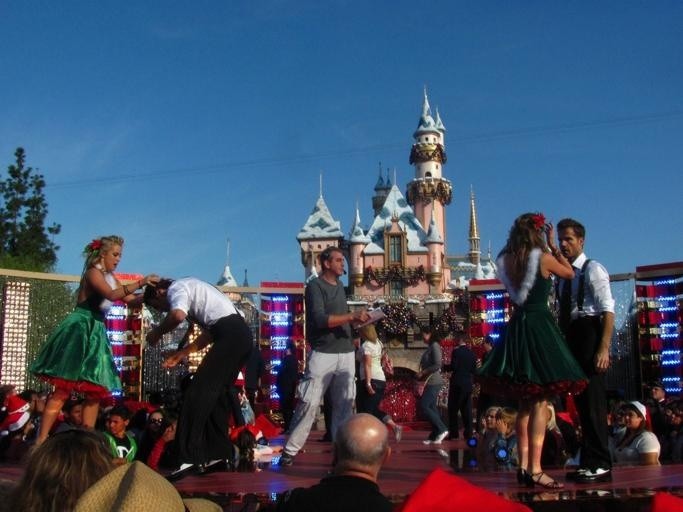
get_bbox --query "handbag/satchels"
[411,379,426,398]
[382,352,394,377]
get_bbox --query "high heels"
[516,469,526,484]
[527,473,564,488]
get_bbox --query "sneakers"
[205,458,230,473]
[165,464,204,480]
[435,431,449,444]
[566,470,586,481]
[577,469,611,483]
[393,425,403,443]
[280,453,296,466]
[423,440,442,445]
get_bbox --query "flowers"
[532,213,546,230]
[83,238,102,257]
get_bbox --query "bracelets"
[122,284,129,296]
[137,280,141,290]
[553,247,561,259]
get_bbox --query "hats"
[0,412,30,435]
[2,396,30,413]
[629,399,653,431]
[62,395,83,411]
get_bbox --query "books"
[351,307,388,331]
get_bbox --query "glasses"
[151,418,163,422]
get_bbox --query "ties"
[559,280,571,335]
[178,317,194,351]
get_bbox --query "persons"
[2,385,252,466]
[473,211,576,490]
[416,325,449,445]
[485,384,683,465]
[21,235,163,466]
[276,343,298,434]
[142,275,254,484]
[358,324,402,442]
[12,426,117,511]
[256,410,402,512]
[475,335,494,432]
[443,330,477,440]
[548,216,617,482]
[275,245,372,470]
